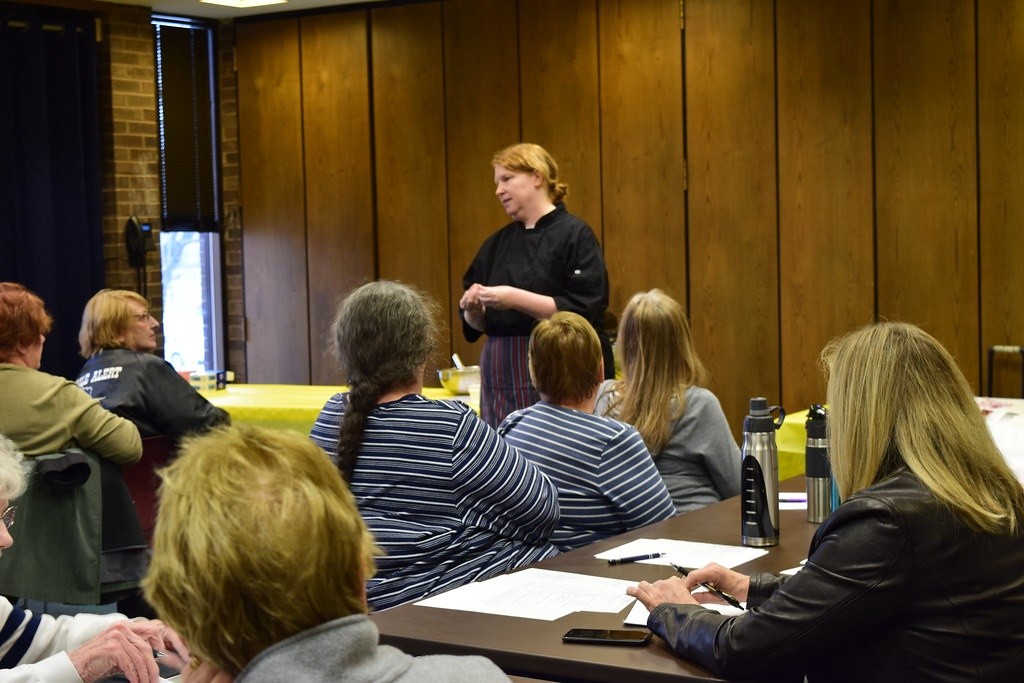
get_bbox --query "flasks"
[804,404,837,524]
[739,397,786,547]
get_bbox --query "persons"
[459,142,616,432]
[1,282,231,683]
[141,422,513,683]
[592,288,741,515]
[494,311,677,552]
[625,321,1024,683]
[305,281,567,616]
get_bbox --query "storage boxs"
[189,370,226,391]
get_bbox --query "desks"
[774,397,1024,482]
[197,384,479,436]
[368,473,822,683]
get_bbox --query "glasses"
[0,506,17,528]
[122,312,151,321]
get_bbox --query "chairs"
[0,436,164,606]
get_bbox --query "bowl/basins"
[437,365,481,395]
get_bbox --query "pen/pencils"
[608,551,668,566]
[154,649,167,659]
[669,562,746,611]
